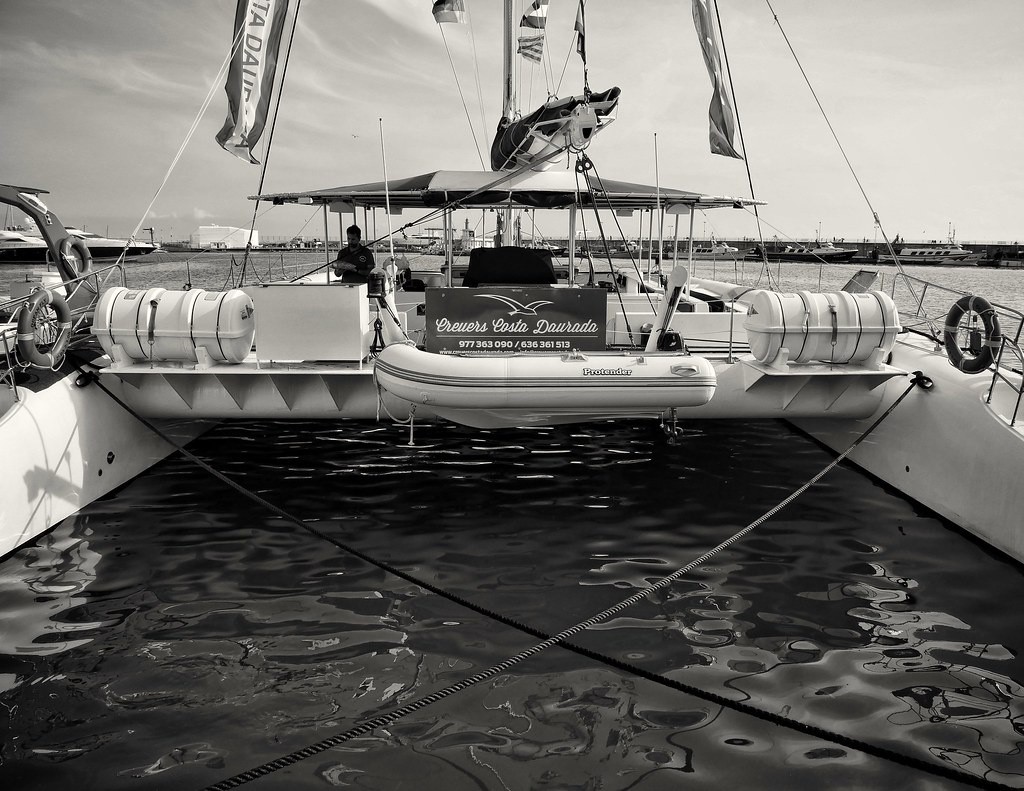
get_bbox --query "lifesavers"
[944,297,998,374]
[18,290,73,370]
[60,236,92,281]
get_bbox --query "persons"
[333,225,375,290]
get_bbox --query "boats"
[0,163,1023,567]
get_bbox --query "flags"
[575,0,597,95]
[689,0,746,163]
[213,0,290,166]
[518,0,551,65]
[432,0,467,26]
